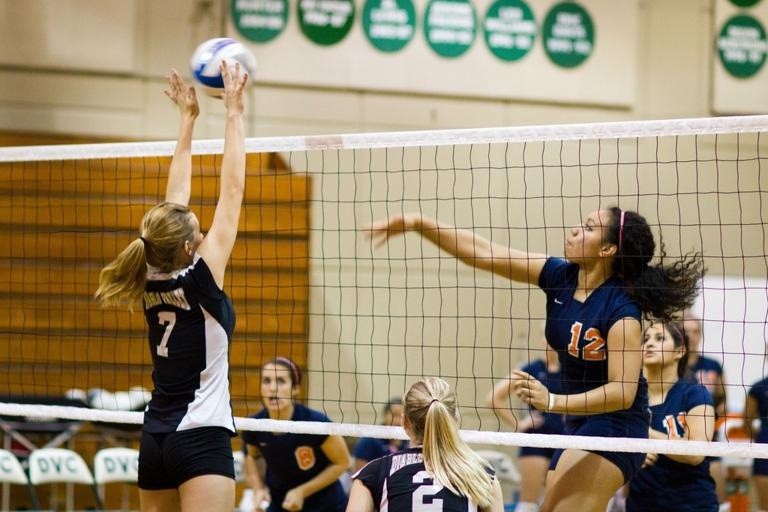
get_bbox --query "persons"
[346,374,504,512]
[92,55,254,511]
[492,343,572,507]
[365,203,710,510]
[238,356,355,512]
[636,310,767,512]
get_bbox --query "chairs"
[1,385,246,512]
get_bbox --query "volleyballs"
[191,37,255,98]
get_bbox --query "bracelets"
[544,394,554,413]
